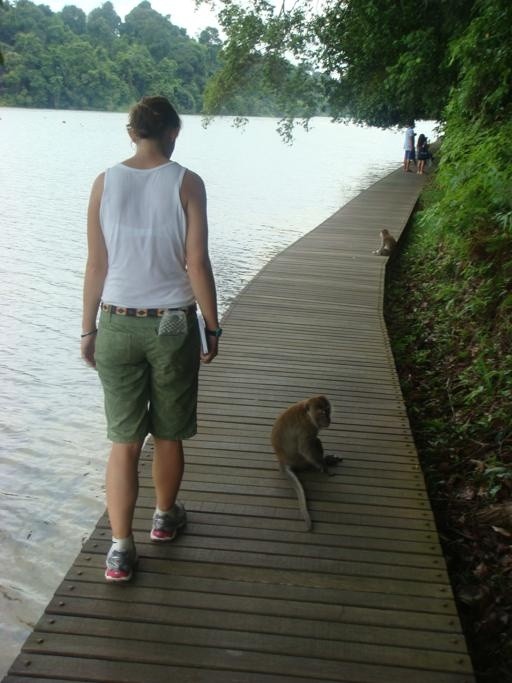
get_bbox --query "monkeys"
[371,229,399,256]
[270,395,343,532]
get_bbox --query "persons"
[80,96,222,582]
[403,122,428,174]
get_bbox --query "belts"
[101,302,198,318]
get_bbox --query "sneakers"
[103,543,139,584]
[150,499,188,543]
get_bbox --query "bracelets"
[205,328,221,337]
[81,329,97,336]
